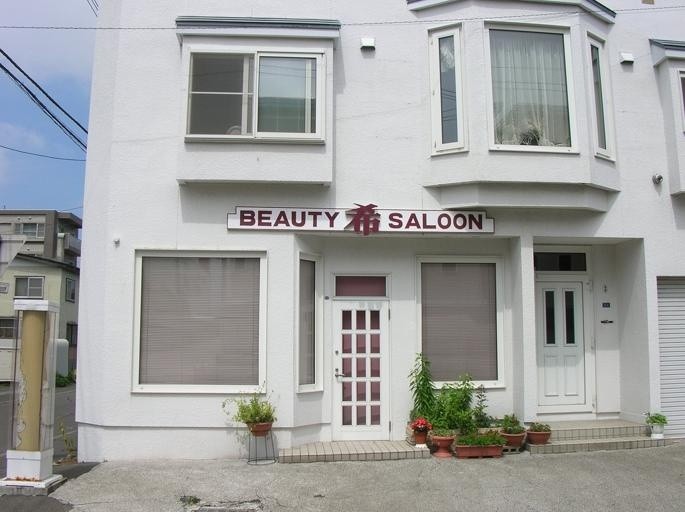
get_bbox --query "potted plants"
[227,389,277,440]
[644,407,669,441]
[404,351,550,460]
